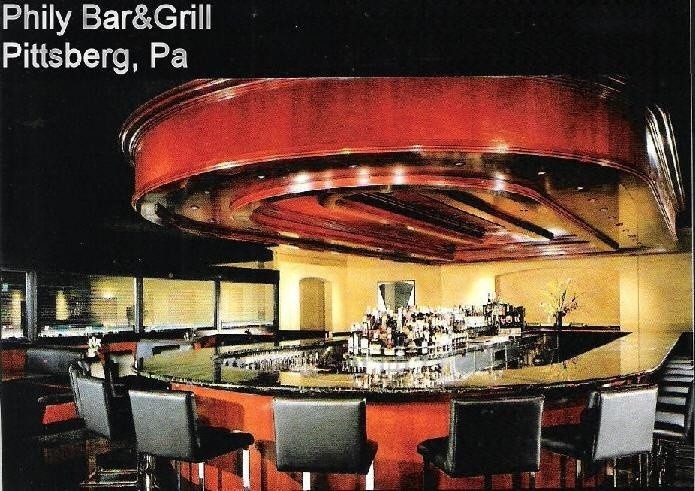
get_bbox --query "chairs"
[69,361,378,491]
[416,355,694,491]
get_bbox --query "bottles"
[343,293,524,357]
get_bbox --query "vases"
[556,312,563,329]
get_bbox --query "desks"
[1,371,53,384]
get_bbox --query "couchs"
[18,348,83,436]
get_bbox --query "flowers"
[540,279,586,317]
